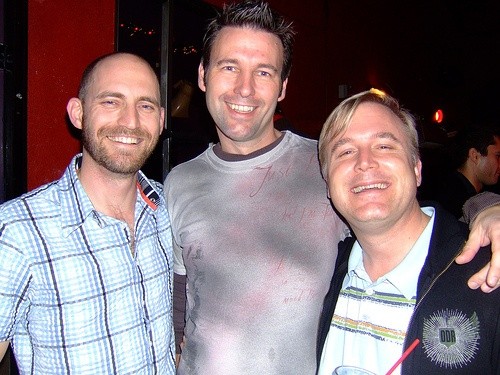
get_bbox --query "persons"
[0,55,180,375]
[427,114,499,211]
[159,2,500,375]
[309,86,500,375]
